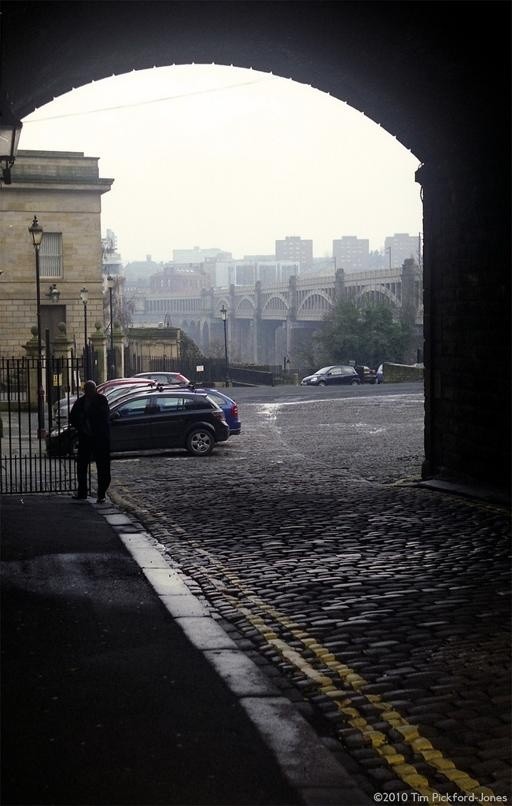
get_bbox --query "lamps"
[44,284,61,303]
[0,102,24,185]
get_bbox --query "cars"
[357,366,376,384]
[41,370,242,457]
[300,364,360,388]
[376,363,383,383]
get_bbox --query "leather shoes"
[72,494,87,499]
[98,497,105,502]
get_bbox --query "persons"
[69,380,111,502]
[376,364,384,384]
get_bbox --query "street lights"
[28,216,47,438]
[220,305,232,388]
[106,274,115,378]
[79,288,94,382]
